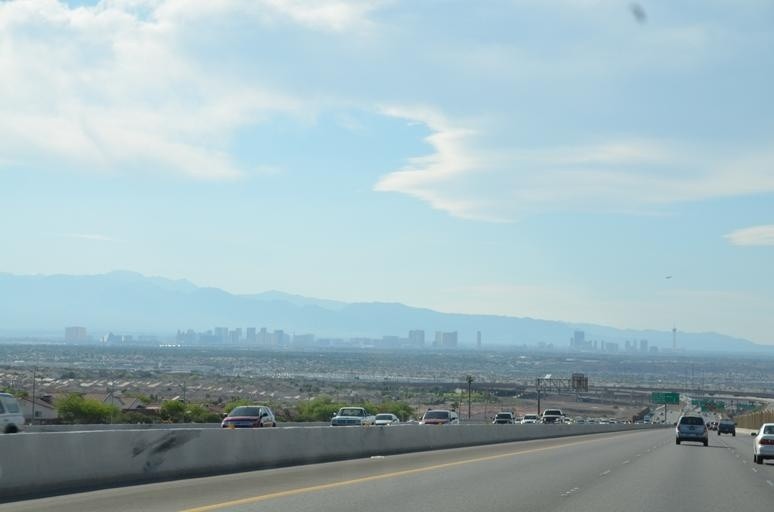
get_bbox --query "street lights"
[466,373,475,420]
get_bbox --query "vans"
[420,408,460,424]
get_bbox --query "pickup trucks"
[331,406,377,425]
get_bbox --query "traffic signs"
[652,392,680,405]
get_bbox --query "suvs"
[0,392,28,434]
[542,408,565,424]
[221,404,278,427]
[494,411,515,424]
[674,414,708,446]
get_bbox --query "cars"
[709,418,737,436]
[561,410,674,425]
[375,413,400,425]
[751,422,774,464]
[520,413,541,424]
